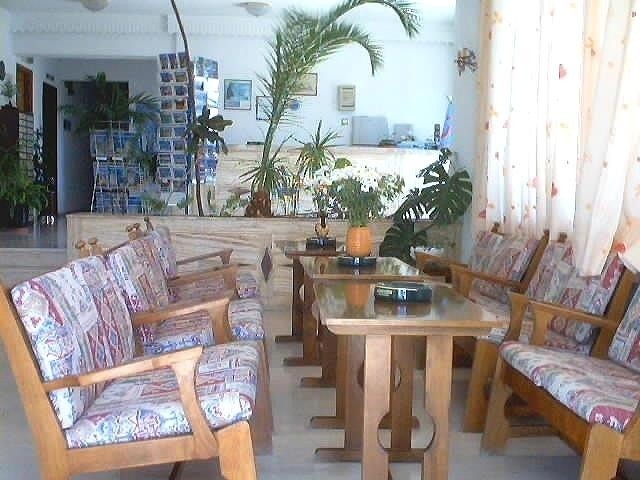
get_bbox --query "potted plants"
[329,171,406,257]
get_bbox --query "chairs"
[0,217,271,479]
[412,223,639,480]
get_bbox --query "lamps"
[247,3,270,17]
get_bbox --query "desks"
[274,240,504,480]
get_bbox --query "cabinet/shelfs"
[0,108,34,225]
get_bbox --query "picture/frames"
[256,96,275,120]
[224,79,252,110]
[273,72,317,96]
